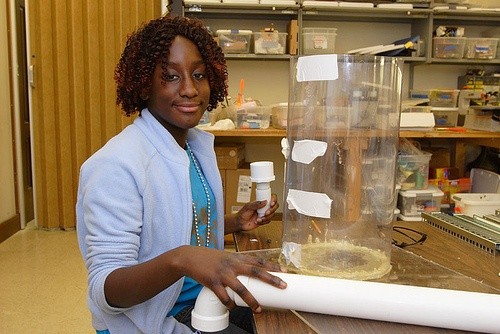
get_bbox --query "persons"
[75,16,300,334]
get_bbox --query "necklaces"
[184,139,212,247]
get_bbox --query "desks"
[234,221,500,334]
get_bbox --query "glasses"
[378,223,427,248]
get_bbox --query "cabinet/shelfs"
[182,0,500,221]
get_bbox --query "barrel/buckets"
[279,53,405,285]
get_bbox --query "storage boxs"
[214,142,257,214]
[433,37,467,58]
[272,105,353,130]
[409,89,461,127]
[238,106,269,129]
[254,32,288,55]
[217,29,253,53]
[397,151,500,217]
[466,37,499,59]
[302,27,338,55]
